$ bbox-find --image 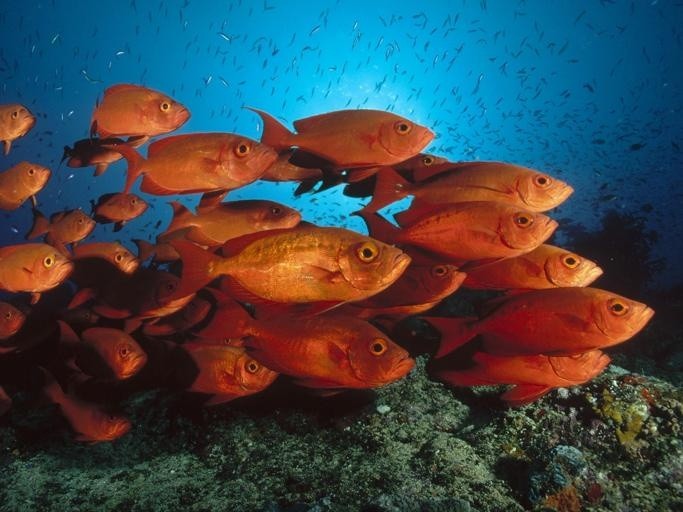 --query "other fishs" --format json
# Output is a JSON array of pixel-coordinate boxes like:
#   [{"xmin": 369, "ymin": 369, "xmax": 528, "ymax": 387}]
[{"xmin": 0, "ymin": 0, "xmax": 683, "ymax": 407}]
